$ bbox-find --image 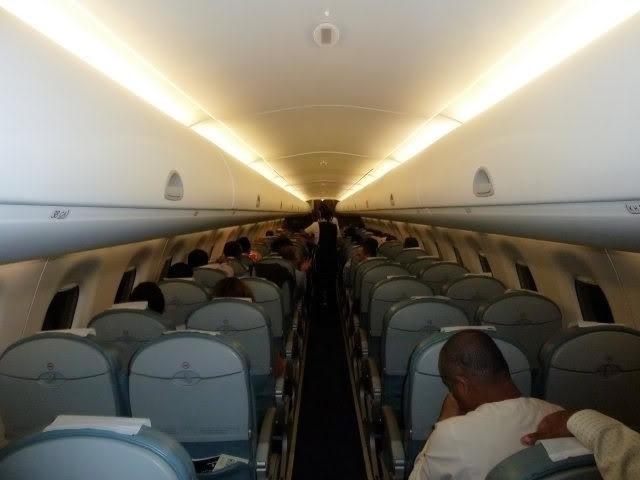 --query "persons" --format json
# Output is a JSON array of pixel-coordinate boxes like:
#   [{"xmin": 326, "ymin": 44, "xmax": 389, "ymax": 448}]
[
  {"xmin": 521, "ymin": 408, "xmax": 640, "ymax": 480},
  {"xmin": 408, "ymin": 328, "xmax": 566, "ymax": 480},
  {"xmin": 126, "ymin": 205, "xmax": 419, "ymax": 330}
]
